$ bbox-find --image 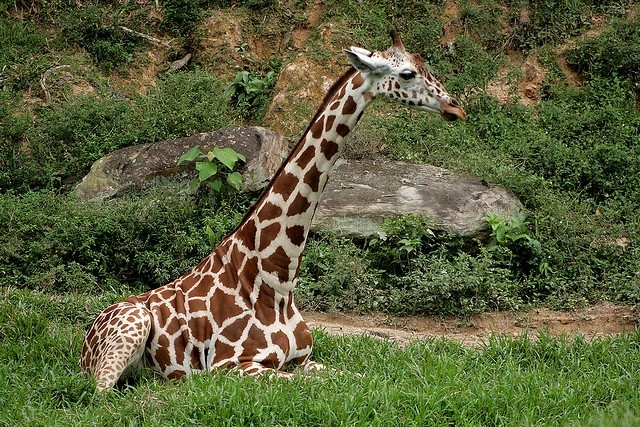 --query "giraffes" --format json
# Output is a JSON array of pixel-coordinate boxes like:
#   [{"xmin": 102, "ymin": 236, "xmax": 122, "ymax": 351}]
[{"xmin": 79, "ymin": 29, "xmax": 467, "ymax": 395}]
